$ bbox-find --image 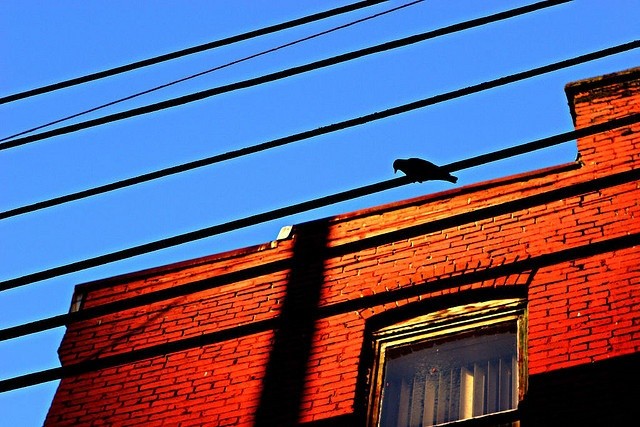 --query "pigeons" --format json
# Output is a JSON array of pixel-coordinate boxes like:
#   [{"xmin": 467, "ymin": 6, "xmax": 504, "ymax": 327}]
[{"xmin": 393, "ymin": 158, "xmax": 458, "ymax": 184}]
